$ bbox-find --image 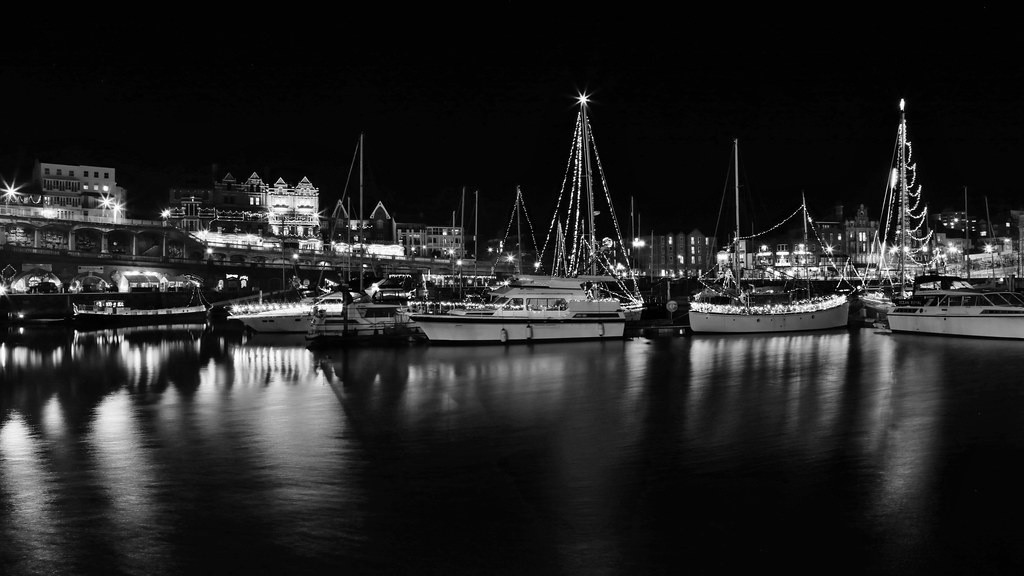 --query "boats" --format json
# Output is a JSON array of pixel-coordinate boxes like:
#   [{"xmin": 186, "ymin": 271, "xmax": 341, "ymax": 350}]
[{"xmin": 0, "ymin": 88, "xmax": 1024, "ymax": 359}]
